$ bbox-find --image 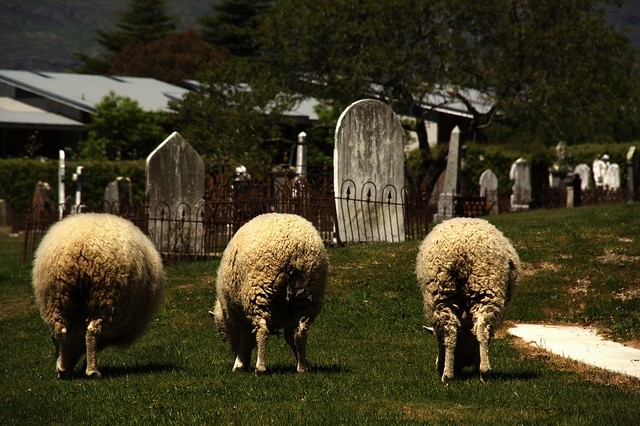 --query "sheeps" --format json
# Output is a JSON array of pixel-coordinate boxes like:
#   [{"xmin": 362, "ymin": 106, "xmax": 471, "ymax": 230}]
[
  {"xmin": 208, "ymin": 212, "xmax": 329, "ymax": 376},
  {"xmin": 415, "ymin": 218, "xmax": 523, "ymax": 382},
  {"xmin": 30, "ymin": 212, "xmax": 166, "ymax": 378}
]
